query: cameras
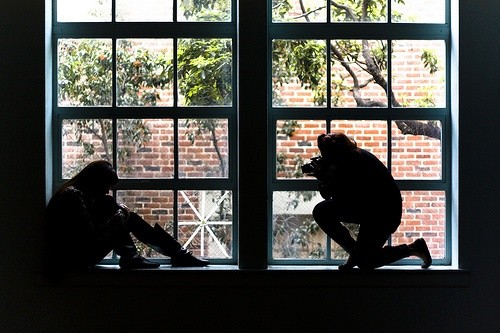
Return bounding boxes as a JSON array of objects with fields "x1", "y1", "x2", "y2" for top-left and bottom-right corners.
[{"x1": 301, "y1": 156, "x2": 322, "y2": 177}]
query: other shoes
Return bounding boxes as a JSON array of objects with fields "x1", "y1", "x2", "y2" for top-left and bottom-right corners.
[
  {"x1": 339, "y1": 250, "x2": 359, "y2": 271},
  {"x1": 413, "y1": 240, "x2": 433, "y2": 270}
]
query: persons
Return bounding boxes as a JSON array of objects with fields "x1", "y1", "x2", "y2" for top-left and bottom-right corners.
[
  {"x1": 313, "y1": 133, "x2": 433, "y2": 270},
  {"x1": 46, "y1": 161, "x2": 210, "y2": 270}
]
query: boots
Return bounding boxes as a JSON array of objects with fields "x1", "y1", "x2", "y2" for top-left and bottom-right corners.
[
  {"x1": 111, "y1": 225, "x2": 160, "y2": 268},
  {"x1": 146, "y1": 223, "x2": 210, "y2": 267}
]
